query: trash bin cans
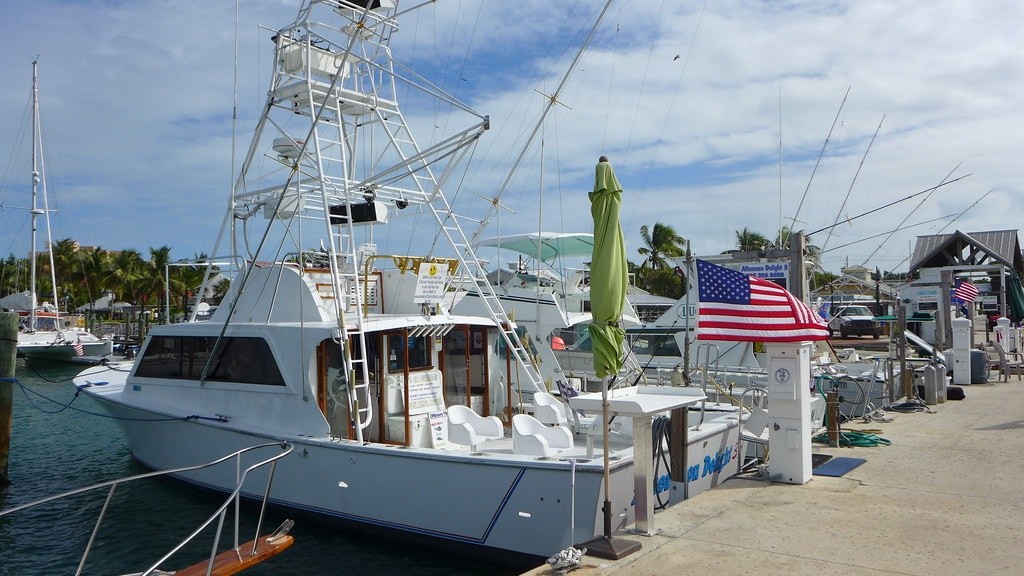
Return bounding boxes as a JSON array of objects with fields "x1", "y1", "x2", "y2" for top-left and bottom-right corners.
[{"x1": 971, "y1": 349, "x2": 987, "y2": 384}]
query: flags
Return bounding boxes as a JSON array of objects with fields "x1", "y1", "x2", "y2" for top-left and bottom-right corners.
[
  {"x1": 954, "y1": 278, "x2": 979, "y2": 304},
  {"x1": 74, "y1": 343, "x2": 83, "y2": 357},
  {"x1": 695, "y1": 258, "x2": 831, "y2": 342}
]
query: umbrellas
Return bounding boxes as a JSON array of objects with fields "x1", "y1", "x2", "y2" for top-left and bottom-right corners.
[
  {"x1": 589, "y1": 161, "x2": 629, "y2": 538},
  {"x1": 1007, "y1": 268, "x2": 1024, "y2": 360}
]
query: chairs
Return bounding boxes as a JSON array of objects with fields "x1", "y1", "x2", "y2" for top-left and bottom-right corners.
[
  {"x1": 571, "y1": 413, "x2": 605, "y2": 459},
  {"x1": 981, "y1": 341, "x2": 1013, "y2": 380},
  {"x1": 445, "y1": 404, "x2": 505, "y2": 453},
  {"x1": 991, "y1": 340, "x2": 1024, "y2": 383},
  {"x1": 533, "y1": 392, "x2": 573, "y2": 428},
  {"x1": 512, "y1": 414, "x2": 574, "y2": 458}
]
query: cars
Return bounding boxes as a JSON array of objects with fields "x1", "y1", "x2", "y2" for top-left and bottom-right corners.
[{"x1": 829, "y1": 302, "x2": 883, "y2": 339}]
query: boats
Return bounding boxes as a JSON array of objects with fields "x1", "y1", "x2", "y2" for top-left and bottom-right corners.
[
  {"x1": 18, "y1": 306, "x2": 114, "y2": 367},
  {"x1": 72, "y1": 0, "x2": 750, "y2": 562},
  {"x1": 436, "y1": 262, "x2": 904, "y2": 459}
]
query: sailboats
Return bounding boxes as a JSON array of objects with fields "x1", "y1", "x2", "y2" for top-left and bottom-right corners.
[{"x1": 0, "y1": 54, "x2": 117, "y2": 371}]
